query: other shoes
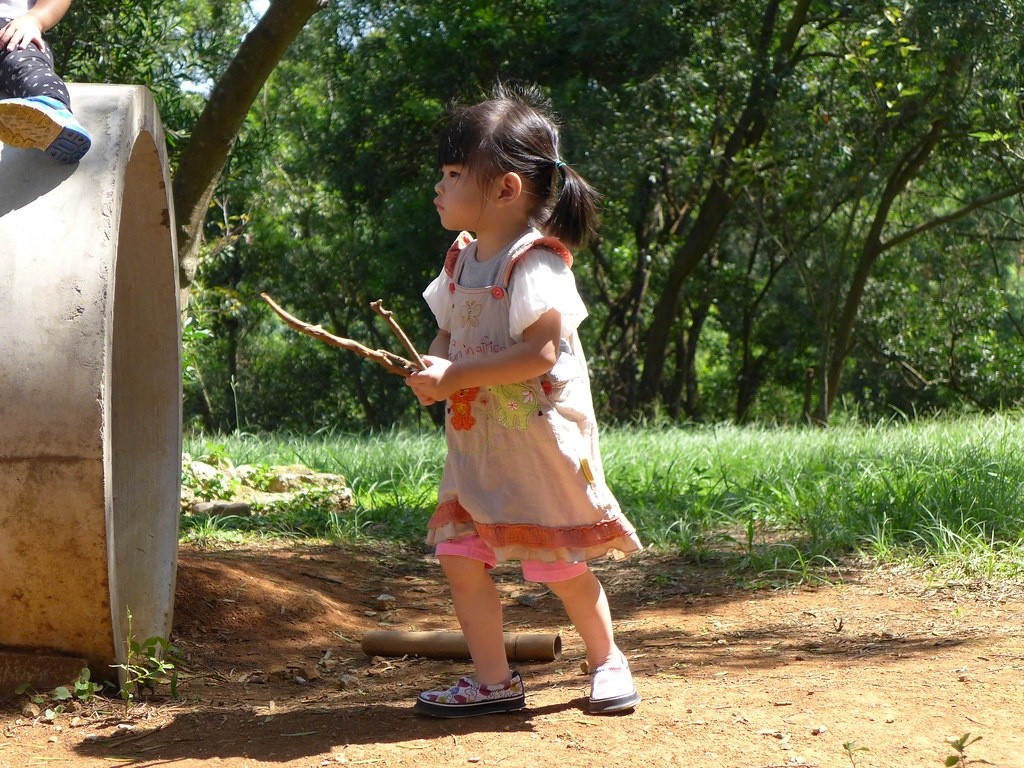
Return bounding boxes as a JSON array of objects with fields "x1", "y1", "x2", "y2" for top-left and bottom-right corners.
[
  {"x1": 587, "y1": 653, "x2": 640, "y2": 714},
  {"x1": 416, "y1": 671, "x2": 527, "y2": 718},
  {"x1": 0, "y1": 95, "x2": 91, "y2": 165}
]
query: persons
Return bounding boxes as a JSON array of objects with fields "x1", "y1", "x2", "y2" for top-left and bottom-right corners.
[
  {"x1": 407, "y1": 80, "x2": 643, "y2": 719},
  {"x1": 0, "y1": 0, "x2": 91, "y2": 164}
]
